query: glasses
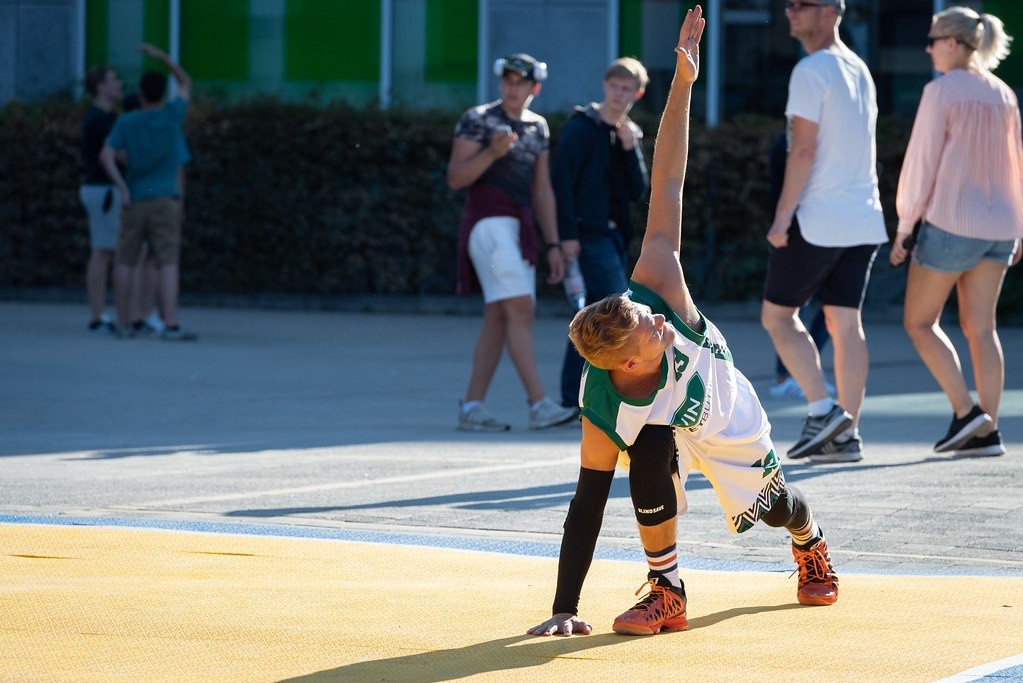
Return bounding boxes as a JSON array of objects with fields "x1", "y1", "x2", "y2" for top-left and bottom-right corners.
[
  {"x1": 785, "y1": 2, "x2": 828, "y2": 11},
  {"x1": 928, "y1": 35, "x2": 955, "y2": 47}
]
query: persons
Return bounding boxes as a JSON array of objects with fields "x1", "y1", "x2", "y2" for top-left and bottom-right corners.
[
  {"x1": 889, "y1": 5, "x2": 1023, "y2": 457},
  {"x1": 99, "y1": 42, "x2": 200, "y2": 341},
  {"x1": 443, "y1": 52, "x2": 577, "y2": 431},
  {"x1": 548, "y1": 53, "x2": 648, "y2": 413},
  {"x1": 765, "y1": 127, "x2": 836, "y2": 402},
  {"x1": 69, "y1": 62, "x2": 124, "y2": 332},
  {"x1": 526, "y1": 5, "x2": 839, "y2": 641},
  {"x1": 758, "y1": 0, "x2": 889, "y2": 462}
]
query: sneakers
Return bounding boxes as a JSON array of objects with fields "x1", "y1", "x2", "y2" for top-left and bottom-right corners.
[
  {"x1": 809, "y1": 438, "x2": 862, "y2": 461},
  {"x1": 527, "y1": 398, "x2": 579, "y2": 431},
  {"x1": 956, "y1": 430, "x2": 1006, "y2": 455},
  {"x1": 769, "y1": 377, "x2": 836, "y2": 400},
  {"x1": 934, "y1": 404, "x2": 991, "y2": 452},
  {"x1": 787, "y1": 405, "x2": 852, "y2": 459},
  {"x1": 458, "y1": 399, "x2": 511, "y2": 432},
  {"x1": 613, "y1": 570, "x2": 688, "y2": 635},
  {"x1": 789, "y1": 526, "x2": 838, "y2": 605}
]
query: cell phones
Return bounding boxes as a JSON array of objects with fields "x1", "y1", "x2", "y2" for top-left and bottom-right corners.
[{"x1": 496, "y1": 125, "x2": 513, "y2": 136}]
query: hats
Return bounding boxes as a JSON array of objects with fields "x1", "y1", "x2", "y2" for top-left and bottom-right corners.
[{"x1": 493, "y1": 53, "x2": 547, "y2": 80}]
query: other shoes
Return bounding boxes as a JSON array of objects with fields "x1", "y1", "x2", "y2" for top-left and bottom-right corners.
[{"x1": 86, "y1": 319, "x2": 197, "y2": 340}]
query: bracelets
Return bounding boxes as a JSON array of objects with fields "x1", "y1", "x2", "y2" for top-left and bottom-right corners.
[{"x1": 549, "y1": 242, "x2": 562, "y2": 250}]
